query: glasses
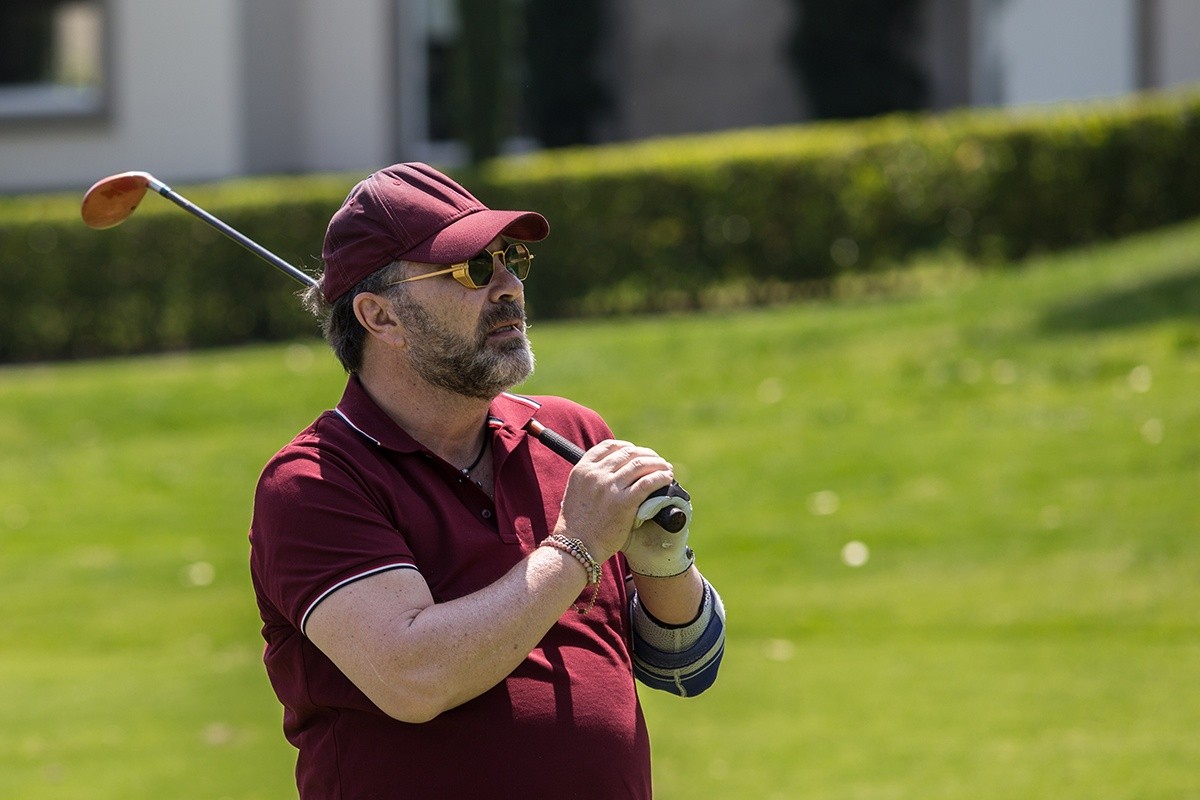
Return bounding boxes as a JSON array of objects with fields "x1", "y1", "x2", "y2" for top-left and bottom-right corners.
[{"x1": 368, "y1": 243, "x2": 534, "y2": 295}]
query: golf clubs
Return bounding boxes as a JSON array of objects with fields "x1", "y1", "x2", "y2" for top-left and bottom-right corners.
[{"x1": 80, "y1": 170, "x2": 688, "y2": 534}]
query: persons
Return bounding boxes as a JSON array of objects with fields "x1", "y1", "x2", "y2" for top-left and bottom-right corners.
[{"x1": 247, "y1": 161, "x2": 725, "y2": 800}]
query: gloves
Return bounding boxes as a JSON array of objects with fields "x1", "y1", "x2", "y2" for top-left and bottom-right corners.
[{"x1": 619, "y1": 478, "x2": 696, "y2": 577}]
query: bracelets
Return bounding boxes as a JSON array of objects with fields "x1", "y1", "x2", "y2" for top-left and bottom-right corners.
[{"x1": 540, "y1": 534, "x2": 603, "y2": 616}]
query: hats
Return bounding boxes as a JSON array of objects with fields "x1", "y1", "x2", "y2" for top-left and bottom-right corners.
[{"x1": 322, "y1": 162, "x2": 549, "y2": 305}]
